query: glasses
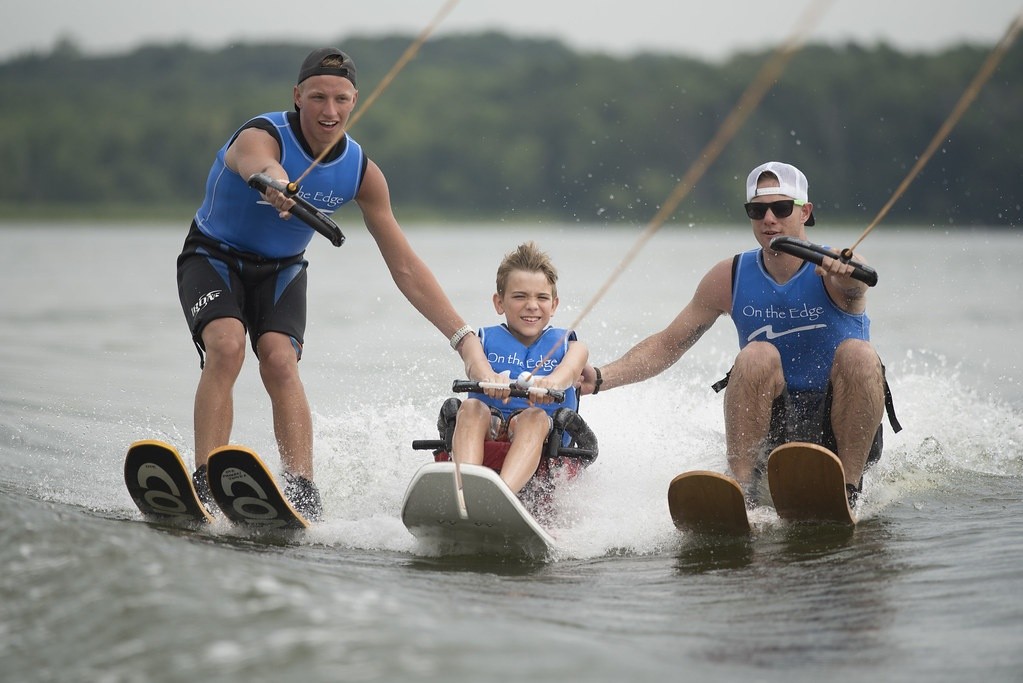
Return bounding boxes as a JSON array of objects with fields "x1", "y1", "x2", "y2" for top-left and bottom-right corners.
[{"x1": 744, "y1": 200, "x2": 807, "y2": 220}]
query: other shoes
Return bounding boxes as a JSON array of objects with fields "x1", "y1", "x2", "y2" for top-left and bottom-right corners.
[
  {"x1": 192, "y1": 464, "x2": 221, "y2": 520},
  {"x1": 280, "y1": 472, "x2": 322, "y2": 522}
]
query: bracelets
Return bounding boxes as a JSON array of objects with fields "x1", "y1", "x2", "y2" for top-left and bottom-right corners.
[{"x1": 593, "y1": 367, "x2": 603, "y2": 396}]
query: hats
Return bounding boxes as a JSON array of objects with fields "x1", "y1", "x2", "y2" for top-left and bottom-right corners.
[
  {"x1": 295, "y1": 47, "x2": 357, "y2": 113},
  {"x1": 746, "y1": 162, "x2": 815, "y2": 226}
]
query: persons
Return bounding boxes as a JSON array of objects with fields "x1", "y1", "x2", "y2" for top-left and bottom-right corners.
[
  {"x1": 450, "y1": 245, "x2": 587, "y2": 497},
  {"x1": 176, "y1": 45, "x2": 476, "y2": 521},
  {"x1": 577, "y1": 162, "x2": 902, "y2": 514}
]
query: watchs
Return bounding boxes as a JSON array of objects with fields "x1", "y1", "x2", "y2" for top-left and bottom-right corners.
[{"x1": 451, "y1": 322, "x2": 475, "y2": 349}]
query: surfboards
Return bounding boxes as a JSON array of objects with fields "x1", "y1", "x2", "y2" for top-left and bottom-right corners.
[
  {"x1": 123, "y1": 439, "x2": 313, "y2": 531},
  {"x1": 668, "y1": 441, "x2": 857, "y2": 535},
  {"x1": 400, "y1": 461, "x2": 557, "y2": 552}
]
